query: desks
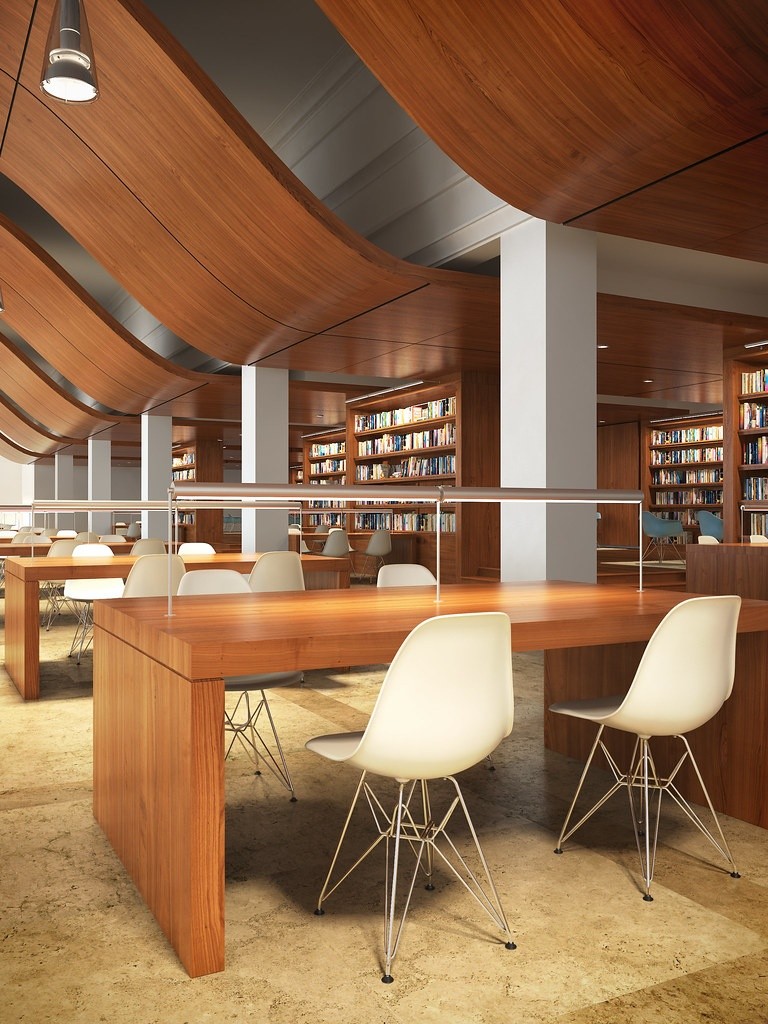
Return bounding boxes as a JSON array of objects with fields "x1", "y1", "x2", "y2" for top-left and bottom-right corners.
[
  {"x1": 302, "y1": 532, "x2": 420, "y2": 585},
  {"x1": 4, "y1": 552, "x2": 351, "y2": 701},
  {"x1": 0, "y1": 541, "x2": 184, "y2": 556},
  {"x1": 0, "y1": 537, "x2": 75, "y2": 543},
  {"x1": 685, "y1": 542, "x2": 768, "y2": 601},
  {"x1": 93, "y1": 579, "x2": 767, "y2": 978}
]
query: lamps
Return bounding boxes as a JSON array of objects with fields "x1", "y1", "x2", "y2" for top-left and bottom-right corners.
[
  {"x1": 37, "y1": 0, "x2": 100, "y2": 105},
  {"x1": 167, "y1": 480, "x2": 442, "y2": 503},
  {"x1": 0, "y1": 500, "x2": 301, "y2": 513},
  {"x1": 345, "y1": 380, "x2": 440, "y2": 403},
  {"x1": 438, "y1": 487, "x2": 645, "y2": 504}
]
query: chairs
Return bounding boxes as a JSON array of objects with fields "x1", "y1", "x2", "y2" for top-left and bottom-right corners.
[
  {"x1": 697, "y1": 511, "x2": 724, "y2": 541},
  {"x1": 641, "y1": 511, "x2": 686, "y2": 565},
  {"x1": 0, "y1": 522, "x2": 767, "y2": 987}
]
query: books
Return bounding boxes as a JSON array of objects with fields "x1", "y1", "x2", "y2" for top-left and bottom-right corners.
[
  {"x1": 741, "y1": 368, "x2": 768, "y2": 394},
  {"x1": 308, "y1": 500, "x2": 346, "y2": 527},
  {"x1": 744, "y1": 512, "x2": 768, "y2": 539},
  {"x1": 172, "y1": 454, "x2": 195, "y2": 525},
  {"x1": 310, "y1": 442, "x2": 346, "y2": 486},
  {"x1": 296, "y1": 470, "x2": 303, "y2": 484},
  {"x1": 740, "y1": 402, "x2": 768, "y2": 429},
  {"x1": 650, "y1": 426, "x2": 723, "y2": 544},
  {"x1": 355, "y1": 484, "x2": 455, "y2": 532},
  {"x1": 742, "y1": 436, "x2": 768, "y2": 465},
  {"x1": 355, "y1": 396, "x2": 456, "y2": 480},
  {"x1": 742, "y1": 476, "x2": 768, "y2": 500}
]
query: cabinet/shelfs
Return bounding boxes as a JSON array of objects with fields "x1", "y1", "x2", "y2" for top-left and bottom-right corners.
[
  {"x1": 303, "y1": 429, "x2": 346, "y2": 554},
  {"x1": 721, "y1": 344, "x2": 768, "y2": 542},
  {"x1": 648, "y1": 414, "x2": 723, "y2": 561},
  {"x1": 344, "y1": 367, "x2": 501, "y2": 585},
  {"x1": 291, "y1": 468, "x2": 303, "y2": 517},
  {"x1": 172, "y1": 440, "x2": 224, "y2": 543}
]
